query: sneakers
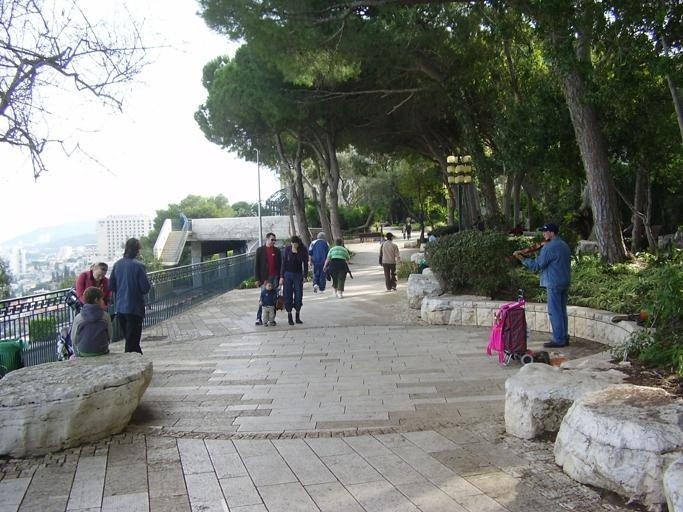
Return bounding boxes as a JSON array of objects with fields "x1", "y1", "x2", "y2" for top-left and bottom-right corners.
[{"x1": 256, "y1": 319, "x2": 275, "y2": 326}]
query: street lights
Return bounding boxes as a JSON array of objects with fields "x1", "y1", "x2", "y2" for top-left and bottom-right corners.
[
  {"x1": 444, "y1": 151, "x2": 474, "y2": 227},
  {"x1": 253, "y1": 144, "x2": 265, "y2": 249}
]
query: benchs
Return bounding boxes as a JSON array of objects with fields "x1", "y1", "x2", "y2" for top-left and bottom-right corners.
[{"x1": 359, "y1": 232, "x2": 384, "y2": 244}]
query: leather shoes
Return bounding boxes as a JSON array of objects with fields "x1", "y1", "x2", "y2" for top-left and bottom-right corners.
[{"x1": 544, "y1": 343, "x2": 564, "y2": 347}]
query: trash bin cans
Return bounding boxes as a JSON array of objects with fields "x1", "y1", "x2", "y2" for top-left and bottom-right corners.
[{"x1": 0, "y1": 339, "x2": 25, "y2": 376}]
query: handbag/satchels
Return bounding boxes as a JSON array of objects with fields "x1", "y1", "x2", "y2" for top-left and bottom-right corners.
[{"x1": 276, "y1": 296, "x2": 284, "y2": 309}]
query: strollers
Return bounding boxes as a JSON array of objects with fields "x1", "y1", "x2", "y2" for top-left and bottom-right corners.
[{"x1": 55, "y1": 312, "x2": 117, "y2": 361}]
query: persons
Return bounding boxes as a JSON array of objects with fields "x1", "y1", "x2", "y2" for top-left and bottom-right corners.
[
  {"x1": 513, "y1": 224, "x2": 571, "y2": 347},
  {"x1": 379, "y1": 233, "x2": 400, "y2": 290},
  {"x1": 253, "y1": 233, "x2": 281, "y2": 325},
  {"x1": 109, "y1": 238, "x2": 151, "y2": 354},
  {"x1": 75, "y1": 262, "x2": 114, "y2": 312},
  {"x1": 323, "y1": 239, "x2": 353, "y2": 298},
  {"x1": 71, "y1": 287, "x2": 112, "y2": 357},
  {"x1": 259, "y1": 280, "x2": 281, "y2": 326},
  {"x1": 279, "y1": 236, "x2": 308, "y2": 325},
  {"x1": 406, "y1": 216, "x2": 412, "y2": 239},
  {"x1": 309, "y1": 232, "x2": 329, "y2": 293}
]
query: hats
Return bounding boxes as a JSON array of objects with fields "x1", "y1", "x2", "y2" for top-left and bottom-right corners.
[{"x1": 539, "y1": 223, "x2": 557, "y2": 232}]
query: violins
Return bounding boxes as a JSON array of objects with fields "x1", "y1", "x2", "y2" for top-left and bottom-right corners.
[{"x1": 503, "y1": 240, "x2": 548, "y2": 263}]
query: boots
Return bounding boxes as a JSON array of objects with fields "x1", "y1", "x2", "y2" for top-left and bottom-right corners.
[
  {"x1": 288, "y1": 314, "x2": 293, "y2": 325},
  {"x1": 296, "y1": 313, "x2": 302, "y2": 323}
]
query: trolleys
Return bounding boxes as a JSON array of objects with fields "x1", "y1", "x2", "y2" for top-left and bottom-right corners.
[{"x1": 490, "y1": 286, "x2": 534, "y2": 367}]
